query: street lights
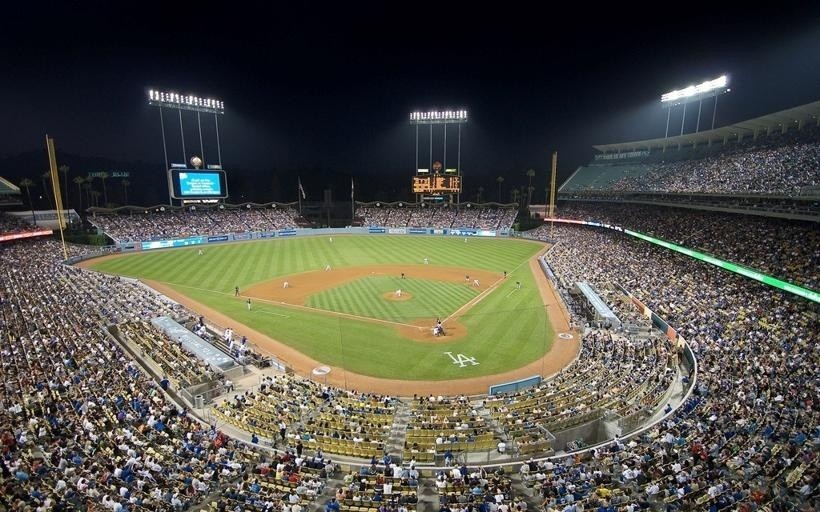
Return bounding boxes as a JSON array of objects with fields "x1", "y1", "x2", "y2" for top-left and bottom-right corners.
[
  {"x1": 409, "y1": 110, "x2": 468, "y2": 204},
  {"x1": 146, "y1": 89, "x2": 226, "y2": 205},
  {"x1": 660, "y1": 74, "x2": 729, "y2": 138}
]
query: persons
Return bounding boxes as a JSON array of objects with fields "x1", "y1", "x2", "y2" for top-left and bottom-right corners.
[
  {"x1": 503, "y1": 270, "x2": 507, "y2": 278},
  {"x1": 516, "y1": 281, "x2": 521, "y2": 290},
  {"x1": 207, "y1": 318, "x2": 547, "y2": 512},
  {"x1": 395, "y1": 287, "x2": 402, "y2": 297},
  {"x1": 401, "y1": 272, "x2": 406, "y2": 279},
  {"x1": 234, "y1": 286, "x2": 241, "y2": 296},
  {"x1": 325, "y1": 264, "x2": 332, "y2": 272},
  {"x1": 465, "y1": 275, "x2": 470, "y2": 287},
  {"x1": 472, "y1": 279, "x2": 480, "y2": 287},
  {"x1": 355, "y1": 199, "x2": 548, "y2": 241},
  {"x1": 0, "y1": 204, "x2": 207, "y2": 511},
  {"x1": 207, "y1": 205, "x2": 302, "y2": 238},
  {"x1": 547, "y1": 129, "x2": 820, "y2": 512},
  {"x1": 246, "y1": 298, "x2": 252, "y2": 311},
  {"x1": 283, "y1": 280, "x2": 288, "y2": 288},
  {"x1": 424, "y1": 256, "x2": 428, "y2": 264}
]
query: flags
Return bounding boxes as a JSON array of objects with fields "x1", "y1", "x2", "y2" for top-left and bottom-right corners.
[{"x1": 299, "y1": 183, "x2": 306, "y2": 199}]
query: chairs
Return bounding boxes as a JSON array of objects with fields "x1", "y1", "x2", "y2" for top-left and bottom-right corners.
[{"x1": 0, "y1": 272, "x2": 820, "y2": 512}]
